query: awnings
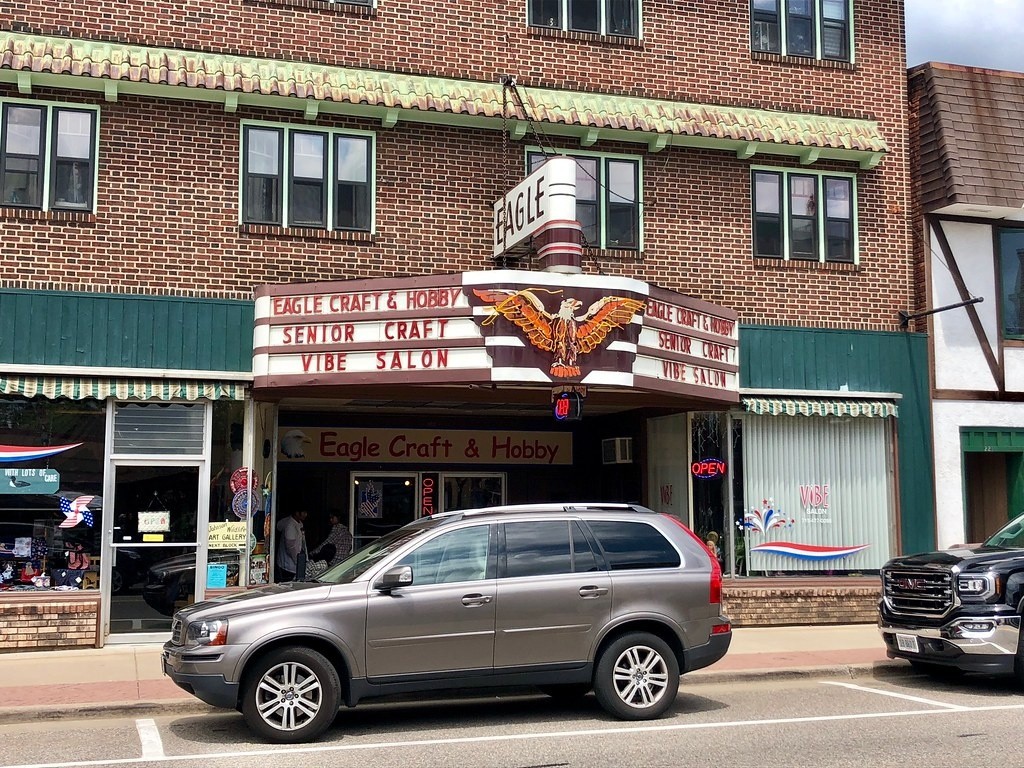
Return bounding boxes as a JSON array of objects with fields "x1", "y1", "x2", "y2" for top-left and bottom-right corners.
[{"x1": 741, "y1": 395, "x2": 898, "y2": 418}]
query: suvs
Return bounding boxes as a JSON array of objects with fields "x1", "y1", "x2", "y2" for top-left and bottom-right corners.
[
  {"x1": 873, "y1": 509, "x2": 1024, "y2": 697},
  {"x1": 158, "y1": 502, "x2": 735, "y2": 746}
]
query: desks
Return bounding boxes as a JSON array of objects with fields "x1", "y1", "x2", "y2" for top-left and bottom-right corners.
[{"x1": 50, "y1": 569, "x2": 97, "y2": 589}]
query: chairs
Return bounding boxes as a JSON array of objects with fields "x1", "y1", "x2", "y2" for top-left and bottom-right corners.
[{"x1": 85, "y1": 556, "x2": 101, "y2": 588}]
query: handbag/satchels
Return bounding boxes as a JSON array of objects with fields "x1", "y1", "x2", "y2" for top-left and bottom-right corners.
[
  {"x1": 68, "y1": 552, "x2": 90, "y2": 569},
  {"x1": 20, "y1": 568, "x2": 40, "y2": 583},
  {"x1": 32, "y1": 573, "x2": 51, "y2": 590},
  {"x1": 137, "y1": 495, "x2": 171, "y2": 532},
  {"x1": 1, "y1": 564, "x2": 17, "y2": 580},
  {"x1": 23, "y1": 561, "x2": 41, "y2": 575}
]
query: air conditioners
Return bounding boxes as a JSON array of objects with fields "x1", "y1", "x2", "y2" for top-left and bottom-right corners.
[{"x1": 602, "y1": 437, "x2": 633, "y2": 464}]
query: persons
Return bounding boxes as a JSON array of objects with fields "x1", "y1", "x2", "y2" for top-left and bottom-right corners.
[
  {"x1": 276, "y1": 503, "x2": 353, "y2": 584},
  {"x1": 706, "y1": 541, "x2": 718, "y2": 556}
]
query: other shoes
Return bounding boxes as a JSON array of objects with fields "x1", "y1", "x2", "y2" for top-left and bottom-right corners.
[{"x1": 72, "y1": 544, "x2": 83, "y2": 551}]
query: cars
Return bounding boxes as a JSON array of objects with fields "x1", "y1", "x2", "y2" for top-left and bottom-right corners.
[
  {"x1": 140, "y1": 537, "x2": 271, "y2": 618},
  {"x1": 0, "y1": 521, "x2": 142, "y2": 597}
]
